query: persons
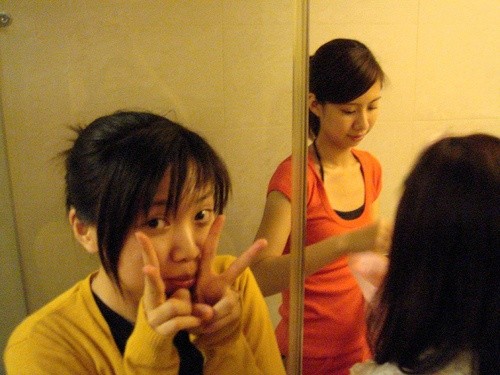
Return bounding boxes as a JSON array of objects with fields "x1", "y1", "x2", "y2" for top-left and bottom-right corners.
[
  {"x1": 249, "y1": 38, "x2": 393, "y2": 375},
  {"x1": 4, "y1": 110, "x2": 287, "y2": 375},
  {"x1": 349, "y1": 133, "x2": 500, "y2": 375}
]
